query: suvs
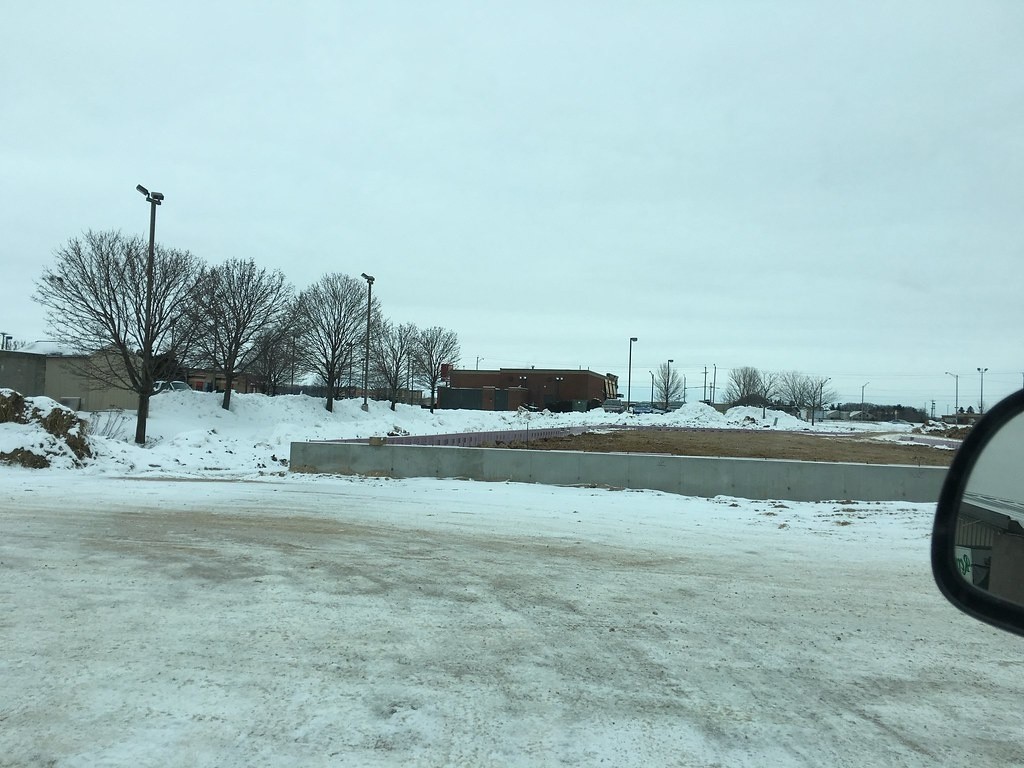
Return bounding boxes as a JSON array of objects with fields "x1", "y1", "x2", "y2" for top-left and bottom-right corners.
[
  {"x1": 602, "y1": 399, "x2": 624, "y2": 414},
  {"x1": 627, "y1": 403, "x2": 636, "y2": 411}
]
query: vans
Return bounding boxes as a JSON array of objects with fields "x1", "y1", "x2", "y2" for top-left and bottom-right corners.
[{"x1": 153, "y1": 380, "x2": 192, "y2": 392}]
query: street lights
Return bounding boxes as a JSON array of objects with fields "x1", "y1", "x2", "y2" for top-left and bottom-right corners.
[
  {"x1": 346, "y1": 342, "x2": 352, "y2": 396},
  {"x1": 361, "y1": 272, "x2": 375, "y2": 412},
  {"x1": 291, "y1": 333, "x2": 301, "y2": 394},
  {"x1": 666, "y1": 360, "x2": 673, "y2": 410},
  {"x1": 6, "y1": 336, "x2": 13, "y2": 350},
  {"x1": 136, "y1": 184, "x2": 164, "y2": 443},
  {"x1": 0, "y1": 332, "x2": 5, "y2": 349},
  {"x1": 628, "y1": 338, "x2": 637, "y2": 411},
  {"x1": 946, "y1": 372, "x2": 958, "y2": 427},
  {"x1": 977, "y1": 368, "x2": 988, "y2": 415}
]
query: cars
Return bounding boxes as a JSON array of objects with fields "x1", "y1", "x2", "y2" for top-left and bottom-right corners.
[
  {"x1": 421, "y1": 398, "x2": 437, "y2": 409},
  {"x1": 633, "y1": 403, "x2": 653, "y2": 414}
]
